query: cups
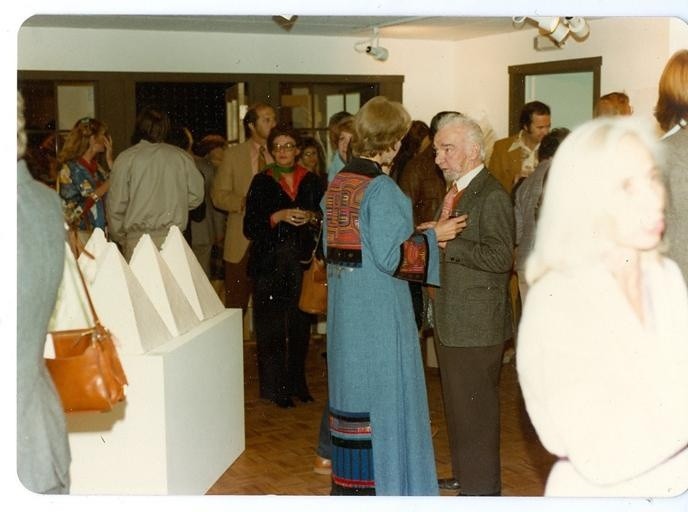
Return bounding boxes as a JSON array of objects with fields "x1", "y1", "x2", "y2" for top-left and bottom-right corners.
[{"x1": 448, "y1": 209, "x2": 463, "y2": 220}]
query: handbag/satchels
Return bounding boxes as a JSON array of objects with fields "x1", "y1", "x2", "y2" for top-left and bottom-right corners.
[
  {"x1": 43, "y1": 323, "x2": 128, "y2": 414},
  {"x1": 297, "y1": 259, "x2": 329, "y2": 316}
]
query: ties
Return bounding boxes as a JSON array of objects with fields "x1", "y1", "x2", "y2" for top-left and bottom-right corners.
[
  {"x1": 428, "y1": 184, "x2": 457, "y2": 300},
  {"x1": 257, "y1": 146, "x2": 267, "y2": 171}
]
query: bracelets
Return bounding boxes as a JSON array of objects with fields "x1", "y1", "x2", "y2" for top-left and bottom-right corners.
[{"x1": 90, "y1": 192, "x2": 100, "y2": 202}]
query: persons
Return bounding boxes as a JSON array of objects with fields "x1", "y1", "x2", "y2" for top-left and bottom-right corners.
[
  {"x1": 488, "y1": 100, "x2": 572, "y2": 304},
  {"x1": 647, "y1": 46, "x2": 686, "y2": 284},
  {"x1": 511, "y1": 116, "x2": 687, "y2": 497},
  {"x1": 313, "y1": 96, "x2": 514, "y2": 495},
  {"x1": 16, "y1": 90, "x2": 74, "y2": 494},
  {"x1": 170, "y1": 104, "x2": 354, "y2": 411},
  {"x1": 54, "y1": 115, "x2": 115, "y2": 259},
  {"x1": 590, "y1": 90, "x2": 634, "y2": 118},
  {"x1": 104, "y1": 102, "x2": 206, "y2": 264}
]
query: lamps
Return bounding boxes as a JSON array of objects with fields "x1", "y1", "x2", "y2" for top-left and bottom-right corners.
[
  {"x1": 512, "y1": 16, "x2": 590, "y2": 44},
  {"x1": 353, "y1": 26, "x2": 389, "y2": 62}
]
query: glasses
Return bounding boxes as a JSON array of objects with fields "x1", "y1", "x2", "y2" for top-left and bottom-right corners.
[
  {"x1": 271, "y1": 142, "x2": 298, "y2": 153},
  {"x1": 302, "y1": 151, "x2": 321, "y2": 160}
]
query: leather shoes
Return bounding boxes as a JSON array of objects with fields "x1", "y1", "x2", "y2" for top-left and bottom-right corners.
[{"x1": 437, "y1": 478, "x2": 460, "y2": 490}]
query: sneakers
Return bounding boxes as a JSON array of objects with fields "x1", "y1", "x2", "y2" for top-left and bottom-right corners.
[{"x1": 313, "y1": 453, "x2": 333, "y2": 476}]
